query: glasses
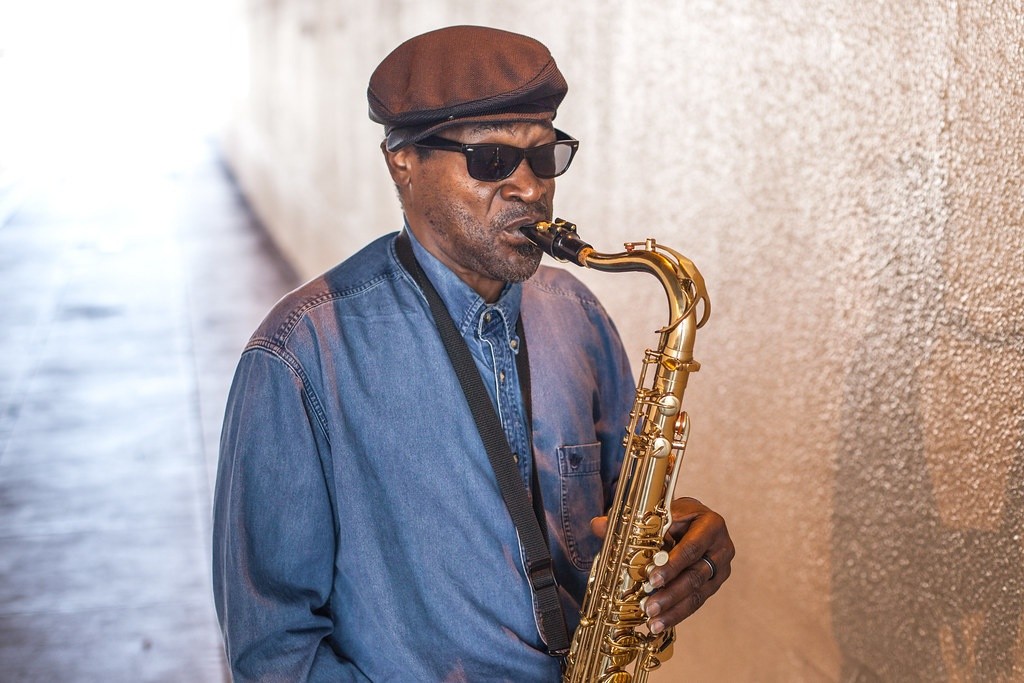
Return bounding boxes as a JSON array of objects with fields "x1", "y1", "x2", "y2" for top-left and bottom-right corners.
[{"x1": 412, "y1": 128, "x2": 579, "y2": 184}]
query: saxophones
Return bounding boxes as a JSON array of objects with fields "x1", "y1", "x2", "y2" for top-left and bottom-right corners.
[{"x1": 516, "y1": 214, "x2": 712, "y2": 683}]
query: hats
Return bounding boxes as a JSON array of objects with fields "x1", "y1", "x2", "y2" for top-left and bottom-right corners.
[{"x1": 365, "y1": 23, "x2": 570, "y2": 130}]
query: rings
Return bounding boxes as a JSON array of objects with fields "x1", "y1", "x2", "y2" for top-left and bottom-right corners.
[{"x1": 701, "y1": 554, "x2": 718, "y2": 581}]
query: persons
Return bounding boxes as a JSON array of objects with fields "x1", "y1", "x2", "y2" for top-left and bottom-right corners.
[{"x1": 212, "y1": 24, "x2": 737, "y2": 682}]
